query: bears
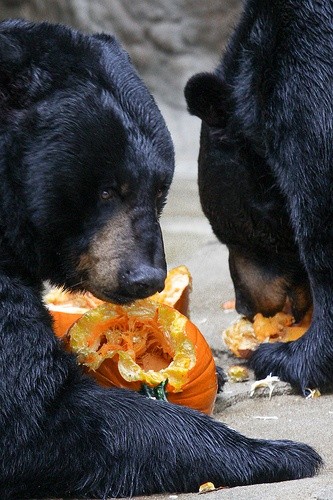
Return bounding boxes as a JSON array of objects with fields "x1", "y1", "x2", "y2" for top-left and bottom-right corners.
[
  {"x1": 0, "y1": 19, "x2": 323, "y2": 500},
  {"x1": 182, "y1": 0, "x2": 333, "y2": 398}
]
